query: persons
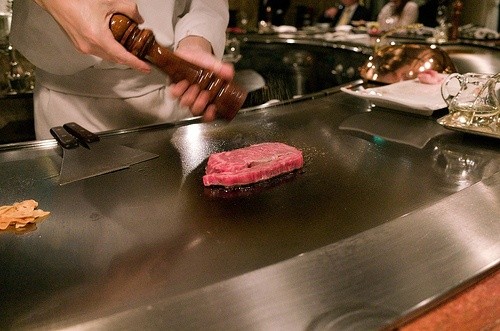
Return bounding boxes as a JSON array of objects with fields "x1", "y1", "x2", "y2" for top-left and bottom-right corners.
[
  {"x1": 317, "y1": 0, "x2": 370, "y2": 30},
  {"x1": 256, "y1": 0, "x2": 290, "y2": 27},
  {"x1": 8, "y1": 0, "x2": 235, "y2": 141},
  {"x1": 376, "y1": 0, "x2": 419, "y2": 30}
]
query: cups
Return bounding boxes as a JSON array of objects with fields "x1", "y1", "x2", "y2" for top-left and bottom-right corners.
[{"x1": 441, "y1": 72, "x2": 500, "y2": 128}]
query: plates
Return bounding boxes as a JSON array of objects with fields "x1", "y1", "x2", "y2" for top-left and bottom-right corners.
[{"x1": 339, "y1": 72, "x2": 459, "y2": 115}]
